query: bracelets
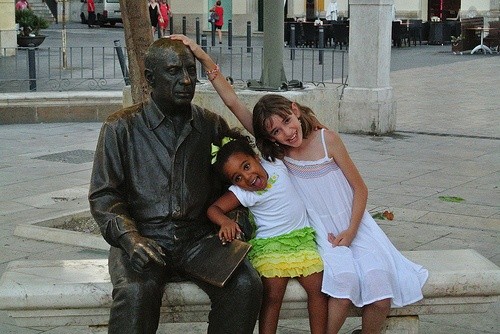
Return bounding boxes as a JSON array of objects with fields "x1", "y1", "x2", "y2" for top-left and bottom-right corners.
[{"x1": 207, "y1": 65, "x2": 220, "y2": 81}]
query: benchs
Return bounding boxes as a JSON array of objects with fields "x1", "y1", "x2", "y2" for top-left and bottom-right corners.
[{"x1": 0, "y1": 248, "x2": 500, "y2": 334}]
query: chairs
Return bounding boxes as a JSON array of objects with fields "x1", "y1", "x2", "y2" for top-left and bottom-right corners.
[{"x1": 284, "y1": 18, "x2": 422, "y2": 50}]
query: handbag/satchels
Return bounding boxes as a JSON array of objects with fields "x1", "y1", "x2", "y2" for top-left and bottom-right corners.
[{"x1": 208, "y1": 11, "x2": 220, "y2": 22}]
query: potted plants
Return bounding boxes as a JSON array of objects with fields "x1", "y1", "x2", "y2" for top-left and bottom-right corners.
[
  {"x1": 451, "y1": 34, "x2": 465, "y2": 53},
  {"x1": 15, "y1": 8, "x2": 47, "y2": 48}
]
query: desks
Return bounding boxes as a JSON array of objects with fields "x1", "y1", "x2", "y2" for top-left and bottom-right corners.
[
  {"x1": 400, "y1": 23, "x2": 415, "y2": 47},
  {"x1": 465, "y1": 27, "x2": 500, "y2": 55},
  {"x1": 323, "y1": 24, "x2": 334, "y2": 47}
]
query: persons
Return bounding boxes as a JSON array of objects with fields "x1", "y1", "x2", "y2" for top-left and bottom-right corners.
[
  {"x1": 209, "y1": 1, "x2": 223, "y2": 44},
  {"x1": 87, "y1": 0, "x2": 95, "y2": 29},
  {"x1": 88, "y1": 38, "x2": 263, "y2": 332},
  {"x1": 147, "y1": 0, "x2": 163, "y2": 36},
  {"x1": 157, "y1": 0, "x2": 173, "y2": 37},
  {"x1": 16, "y1": 0, "x2": 29, "y2": 14},
  {"x1": 165, "y1": 34, "x2": 394, "y2": 334},
  {"x1": 206, "y1": 136, "x2": 329, "y2": 334}
]
